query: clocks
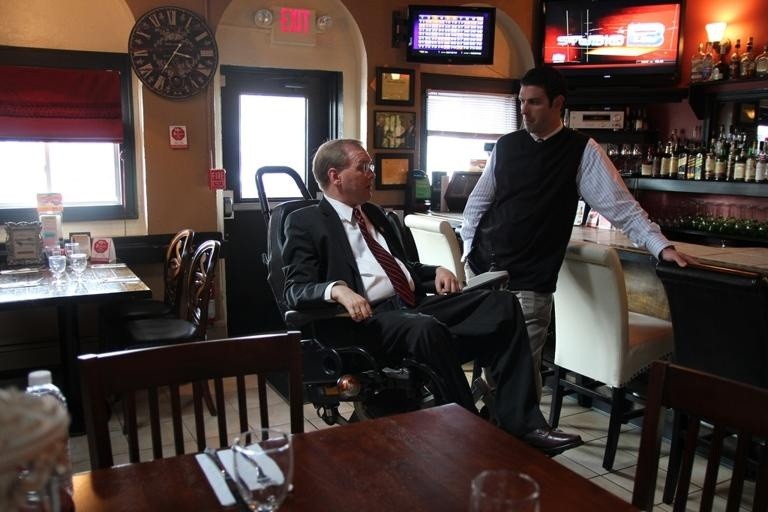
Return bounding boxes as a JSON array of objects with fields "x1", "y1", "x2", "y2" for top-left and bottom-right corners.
[{"x1": 127, "y1": 5, "x2": 220, "y2": 102}]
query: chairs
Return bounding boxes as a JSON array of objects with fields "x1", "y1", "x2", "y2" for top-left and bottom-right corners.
[
  {"x1": 404, "y1": 212, "x2": 511, "y2": 294},
  {"x1": 108, "y1": 240, "x2": 221, "y2": 433},
  {"x1": 121, "y1": 229, "x2": 195, "y2": 319},
  {"x1": 548, "y1": 241, "x2": 677, "y2": 470},
  {"x1": 78, "y1": 331, "x2": 303, "y2": 465},
  {"x1": 631, "y1": 360, "x2": 768, "y2": 512}
]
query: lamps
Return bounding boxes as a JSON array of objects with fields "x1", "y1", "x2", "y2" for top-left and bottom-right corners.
[
  {"x1": 705, "y1": 22, "x2": 727, "y2": 44},
  {"x1": 555, "y1": 8, "x2": 627, "y2": 47}
]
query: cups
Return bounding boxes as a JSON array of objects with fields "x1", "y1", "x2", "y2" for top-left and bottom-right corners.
[
  {"x1": 49, "y1": 255, "x2": 66, "y2": 292},
  {"x1": 64, "y1": 243, "x2": 80, "y2": 274},
  {"x1": 469, "y1": 469, "x2": 540, "y2": 512},
  {"x1": 45, "y1": 245, "x2": 61, "y2": 258},
  {"x1": 70, "y1": 254, "x2": 88, "y2": 294},
  {"x1": 231, "y1": 427, "x2": 296, "y2": 512}
]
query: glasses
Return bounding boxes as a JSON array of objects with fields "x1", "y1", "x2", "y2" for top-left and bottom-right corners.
[{"x1": 336, "y1": 160, "x2": 376, "y2": 174}]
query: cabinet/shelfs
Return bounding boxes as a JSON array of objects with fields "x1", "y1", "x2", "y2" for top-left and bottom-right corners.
[{"x1": 398, "y1": 0, "x2": 768, "y2": 436}]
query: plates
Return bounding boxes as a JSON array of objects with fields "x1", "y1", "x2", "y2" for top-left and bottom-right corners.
[{"x1": 194, "y1": 442, "x2": 294, "y2": 507}]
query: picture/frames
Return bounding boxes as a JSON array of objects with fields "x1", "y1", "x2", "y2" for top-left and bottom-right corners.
[
  {"x1": 376, "y1": 67, "x2": 415, "y2": 105},
  {"x1": 375, "y1": 154, "x2": 413, "y2": 191},
  {"x1": 374, "y1": 111, "x2": 417, "y2": 148}
]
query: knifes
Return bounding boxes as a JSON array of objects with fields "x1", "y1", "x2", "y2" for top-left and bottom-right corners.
[{"x1": 203, "y1": 445, "x2": 253, "y2": 511}]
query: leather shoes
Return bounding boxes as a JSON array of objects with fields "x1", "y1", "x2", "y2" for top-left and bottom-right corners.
[{"x1": 521, "y1": 427, "x2": 584, "y2": 454}]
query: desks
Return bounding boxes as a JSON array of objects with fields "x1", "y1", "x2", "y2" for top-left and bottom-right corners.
[
  {"x1": 0, "y1": 251, "x2": 151, "y2": 400},
  {"x1": 67, "y1": 403, "x2": 645, "y2": 512}
]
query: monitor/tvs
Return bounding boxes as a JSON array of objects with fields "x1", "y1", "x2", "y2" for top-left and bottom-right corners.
[
  {"x1": 537, "y1": 2, "x2": 686, "y2": 79},
  {"x1": 403, "y1": 5, "x2": 496, "y2": 65}
]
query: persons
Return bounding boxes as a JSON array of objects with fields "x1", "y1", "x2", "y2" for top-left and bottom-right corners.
[
  {"x1": 459, "y1": 67, "x2": 701, "y2": 423},
  {"x1": 283, "y1": 140, "x2": 585, "y2": 454}
]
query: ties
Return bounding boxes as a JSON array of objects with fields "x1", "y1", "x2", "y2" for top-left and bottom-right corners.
[{"x1": 352, "y1": 207, "x2": 417, "y2": 309}]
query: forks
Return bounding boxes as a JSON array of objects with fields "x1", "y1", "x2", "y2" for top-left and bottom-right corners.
[{"x1": 232, "y1": 445, "x2": 283, "y2": 497}]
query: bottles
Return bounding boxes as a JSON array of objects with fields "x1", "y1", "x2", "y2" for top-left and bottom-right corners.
[
  {"x1": 660, "y1": 143, "x2": 671, "y2": 177},
  {"x1": 734, "y1": 148, "x2": 744, "y2": 183},
  {"x1": 635, "y1": 109, "x2": 644, "y2": 177},
  {"x1": 669, "y1": 150, "x2": 677, "y2": 178},
  {"x1": 741, "y1": 37, "x2": 756, "y2": 80},
  {"x1": 653, "y1": 141, "x2": 662, "y2": 177},
  {"x1": 640, "y1": 146, "x2": 653, "y2": 177},
  {"x1": 711, "y1": 42, "x2": 729, "y2": 84},
  {"x1": 729, "y1": 39, "x2": 741, "y2": 82},
  {"x1": 702, "y1": 42, "x2": 714, "y2": 83},
  {"x1": 715, "y1": 148, "x2": 726, "y2": 181},
  {"x1": 690, "y1": 43, "x2": 705, "y2": 84},
  {"x1": 687, "y1": 146, "x2": 695, "y2": 180},
  {"x1": 678, "y1": 145, "x2": 688, "y2": 179},
  {"x1": 745, "y1": 147, "x2": 756, "y2": 183},
  {"x1": 755, "y1": 152, "x2": 768, "y2": 183},
  {"x1": 705, "y1": 149, "x2": 715, "y2": 180},
  {"x1": 755, "y1": 45, "x2": 768, "y2": 79},
  {"x1": 664, "y1": 124, "x2": 768, "y2": 150},
  {"x1": 607, "y1": 128, "x2": 621, "y2": 174},
  {"x1": 622, "y1": 107, "x2": 634, "y2": 177},
  {"x1": 24, "y1": 370, "x2": 74, "y2": 510},
  {"x1": 725, "y1": 144, "x2": 734, "y2": 181},
  {"x1": 695, "y1": 146, "x2": 704, "y2": 181}
]
